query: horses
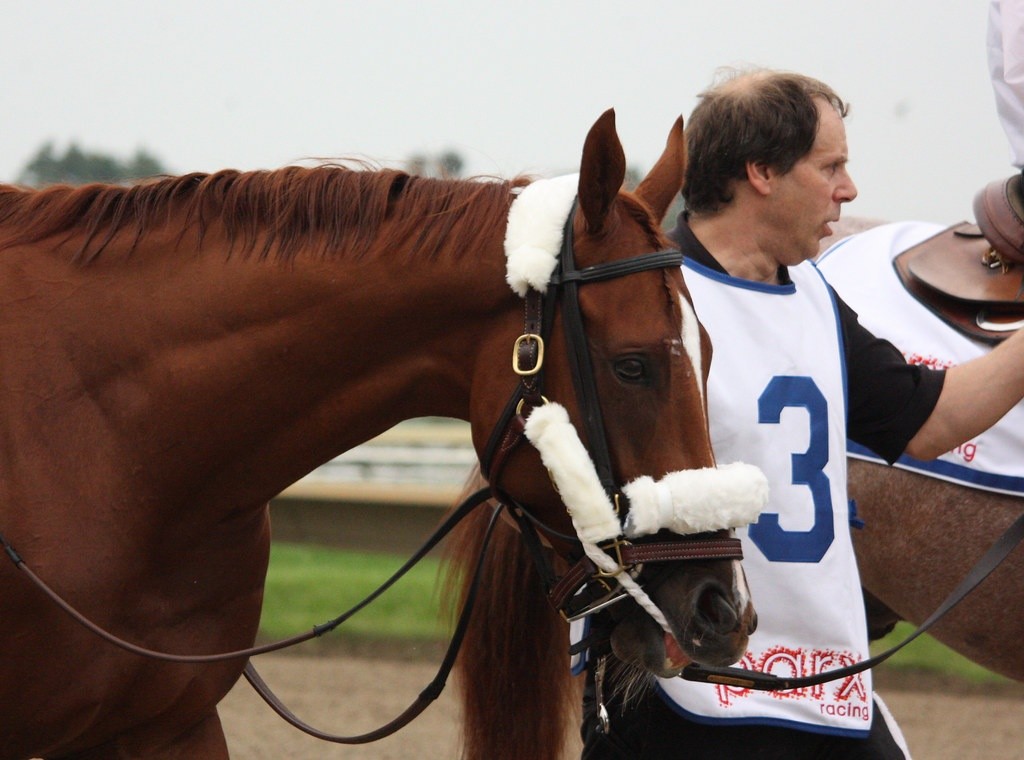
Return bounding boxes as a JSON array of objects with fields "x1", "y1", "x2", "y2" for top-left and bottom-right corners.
[
  {"x1": 0, "y1": 110, "x2": 757, "y2": 759},
  {"x1": 430, "y1": 215, "x2": 1023, "y2": 760}
]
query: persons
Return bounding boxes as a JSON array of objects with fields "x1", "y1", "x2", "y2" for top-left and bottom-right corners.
[
  {"x1": 566, "y1": 65, "x2": 1023, "y2": 760},
  {"x1": 404, "y1": 146, "x2": 463, "y2": 180}
]
